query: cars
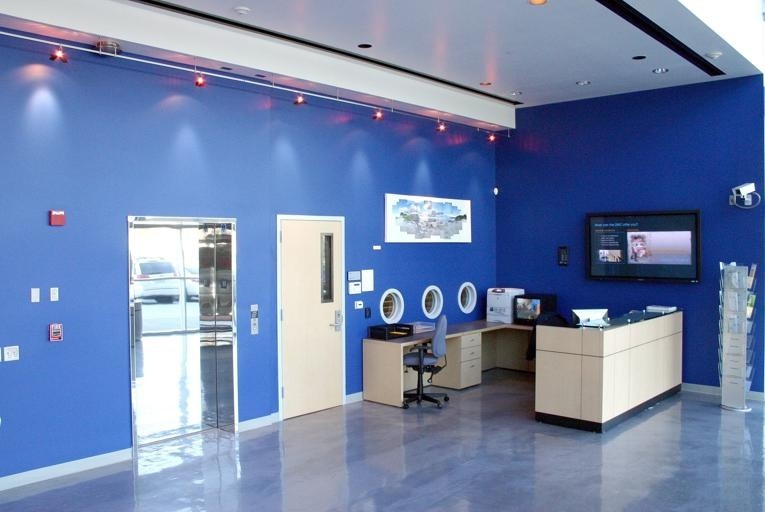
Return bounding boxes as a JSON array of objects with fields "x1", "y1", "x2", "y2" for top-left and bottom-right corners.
[{"x1": 130, "y1": 256, "x2": 199, "y2": 302}]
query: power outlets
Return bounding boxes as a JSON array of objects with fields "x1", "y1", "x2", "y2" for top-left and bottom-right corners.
[{"x1": 3, "y1": 345, "x2": 19, "y2": 362}]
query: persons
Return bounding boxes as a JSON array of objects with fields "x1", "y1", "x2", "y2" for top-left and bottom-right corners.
[{"x1": 630, "y1": 236, "x2": 641, "y2": 261}]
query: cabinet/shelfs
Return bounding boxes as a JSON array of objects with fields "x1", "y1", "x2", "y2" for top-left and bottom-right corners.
[
  {"x1": 361, "y1": 324, "x2": 533, "y2": 408},
  {"x1": 534, "y1": 308, "x2": 684, "y2": 433}
]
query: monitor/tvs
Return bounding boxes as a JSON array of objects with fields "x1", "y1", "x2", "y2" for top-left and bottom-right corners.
[
  {"x1": 586, "y1": 208, "x2": 702, "y2": 288},
  {"x1": 514, "y1": 294, "x2": 557, "y2": 326}
]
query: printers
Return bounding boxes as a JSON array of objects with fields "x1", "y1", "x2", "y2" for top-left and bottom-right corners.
[{"x1": 486, "y1": 287, "x2": 524, "y2": 324}]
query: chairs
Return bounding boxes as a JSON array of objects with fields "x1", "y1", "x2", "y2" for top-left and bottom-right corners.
[
  {"x1": 537, "y1": 314, "x2": 570, "y2": 328},
  {"x1": 401, "y1": 314, "x2": 451, "y2": 411}
]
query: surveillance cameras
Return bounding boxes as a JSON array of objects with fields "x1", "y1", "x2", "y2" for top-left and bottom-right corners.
[{"x1": 732, "y1": 183, "x2": 756, "y2": 197}]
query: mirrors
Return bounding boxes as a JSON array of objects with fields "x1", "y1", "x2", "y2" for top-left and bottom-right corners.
[{"x1": 125, "y1": 214, "x2": 242, "y2": 464}]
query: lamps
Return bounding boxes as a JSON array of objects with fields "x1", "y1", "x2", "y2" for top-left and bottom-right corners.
[
  {"x1": 193, "y1": 73, "x2": 208, "y2": 89},
  {"x1": 486, "y1": 132, "x2": 496, "y2": 144},
  {"x1": 372, "y1": 107, "x2": 385, "y2": 121},
  {"x1": 436, "y1": 122, "x2": 447, "y2": 133},
  {"x1": 46, "y1": 43, "x2": 69, "y2": 66},
  {"x1": 293, "y1": 91, "x2": 307, "y2": 108}
]
